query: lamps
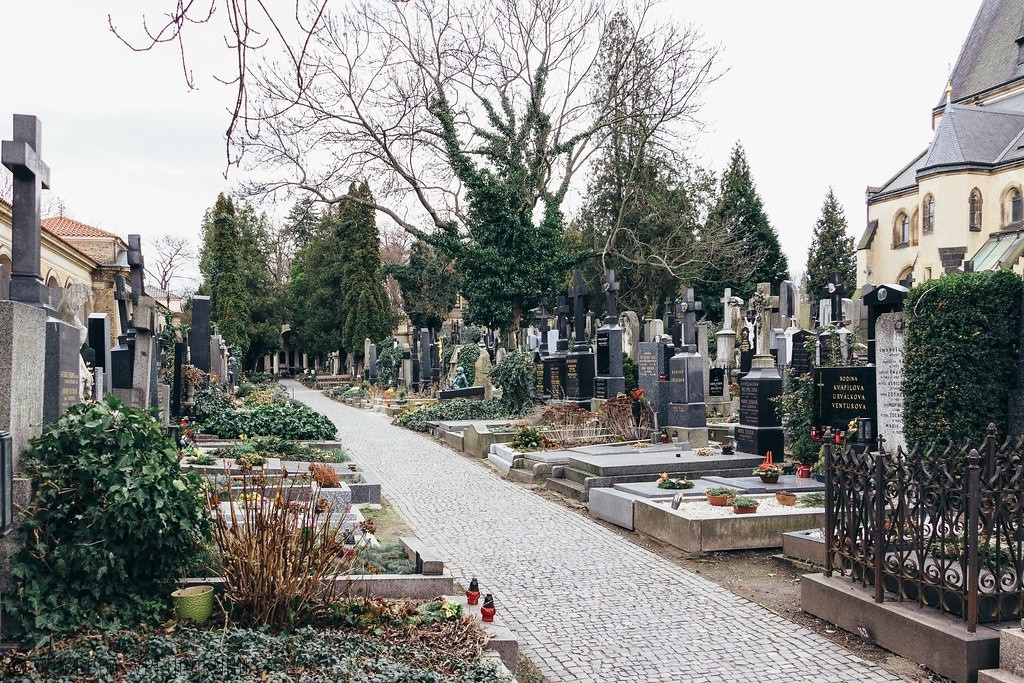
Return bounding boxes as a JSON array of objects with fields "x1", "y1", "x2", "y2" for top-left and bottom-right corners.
[{"x1": 857, "y1": 418, "x2": 873, "y2": 443}]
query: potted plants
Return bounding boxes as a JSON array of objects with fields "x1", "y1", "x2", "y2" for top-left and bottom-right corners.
[
  {"x1": 706, "y1": 487, "x2": 737, "y2": 507},
  {"x1": 791, "y1": 438, "x2": 816, "y2": 478},
  {"x1": 730, "y1": 497, "x2": 759, "y2": 514},
  {"x1": 812, "y1": 458, "x2": 826, "y2": 481}
]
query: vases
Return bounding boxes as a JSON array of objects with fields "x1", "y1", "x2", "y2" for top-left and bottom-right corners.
[
  {"x1": 761, "y1": 475, "x2": 779, "y2": 483},
  {"x1": 171, "y1": 585, "x2": 213, "y2": 623}
]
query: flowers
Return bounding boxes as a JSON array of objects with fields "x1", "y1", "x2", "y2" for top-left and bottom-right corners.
[{"x1": 753, "y1": 466, "x2": 783, "y2": 479}]
